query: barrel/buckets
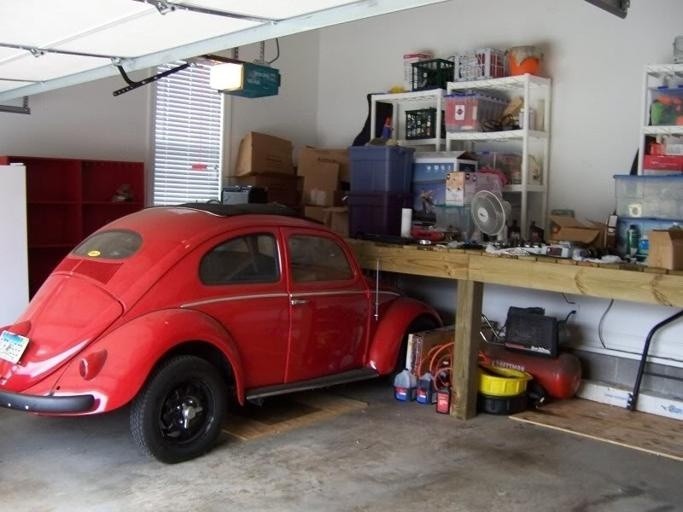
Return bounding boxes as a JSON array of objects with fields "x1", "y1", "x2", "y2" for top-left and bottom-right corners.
[
  {"x1": 393, "y1": 368, "x2": 450, "y2": 414},
  {"x1": 503, "y1": 45, "x2": 542, "y2": 75}
]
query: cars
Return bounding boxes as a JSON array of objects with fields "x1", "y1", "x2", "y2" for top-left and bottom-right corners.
[{"x1": 1, "y1": 200, "x2": 443, "y2": 463}]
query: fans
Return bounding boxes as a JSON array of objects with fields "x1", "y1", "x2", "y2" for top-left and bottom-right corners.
[{"x1": 470, "y1": 189, "x2": 513, "y2": 248}]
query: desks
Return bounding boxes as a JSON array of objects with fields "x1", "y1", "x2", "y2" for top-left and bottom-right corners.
[{"x1": 290, "y1": 239, "x2": 682, "y2": 421}]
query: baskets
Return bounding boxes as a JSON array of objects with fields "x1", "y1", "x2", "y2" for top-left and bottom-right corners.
[
  {"x1": 405, "y1": 109, "x2": 445, "y2": 139},
  {"x1": 454, "y1": 47, "x2": 506, "y2": 82},
  {"x1": 411, "y1": 58, "x2": 453, "y2": 91}
]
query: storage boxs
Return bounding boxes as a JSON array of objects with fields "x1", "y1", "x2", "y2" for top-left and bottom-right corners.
[
  {"x1": 649, "y1": 86, "x2": 683, "y2": 126},
  {"x1": 235, "y1": 131, "x2": 350, "y2": 238},
  {"x1": 549, "y1": 175, "x2": 683, "y2": 270},
  {"x1": 349, "y1": 192, "x2": 413, "y2": 242},
  {"x1": 444, "y1": 91, "x2": 507, "y2": 132},
  {"x1": 411, "y1": 151, "x2": 478, "y2": 224},
  {"x1": 348, "y1": 145, "x2": 416, "y2": 192}
]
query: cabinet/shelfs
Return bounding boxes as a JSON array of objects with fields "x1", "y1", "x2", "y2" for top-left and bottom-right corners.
[
  {"x1": 370, "y1": 87, "x2": 447, "y2": 151},
  {"x1": 445, "y1": 73, "x2": 552, "y2": 242},
  {"x1": 637, "y1": 64, "x2": 683, "y2": 175},
  {"x1": 0, "y1": 155, "x2": 145, "y2": 301}
]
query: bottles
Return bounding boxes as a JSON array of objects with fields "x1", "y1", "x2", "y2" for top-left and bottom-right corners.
[
  {"x1": 628, "y1": 225, "x2": 638, "y2": 255},
  {"x1": 518, "y1": 106, "x2": 535, "y2": 131}
]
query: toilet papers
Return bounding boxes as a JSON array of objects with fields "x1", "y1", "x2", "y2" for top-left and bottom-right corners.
[{"x1": 401, "y1": 208, "x2": 413, "y2": 238}]
